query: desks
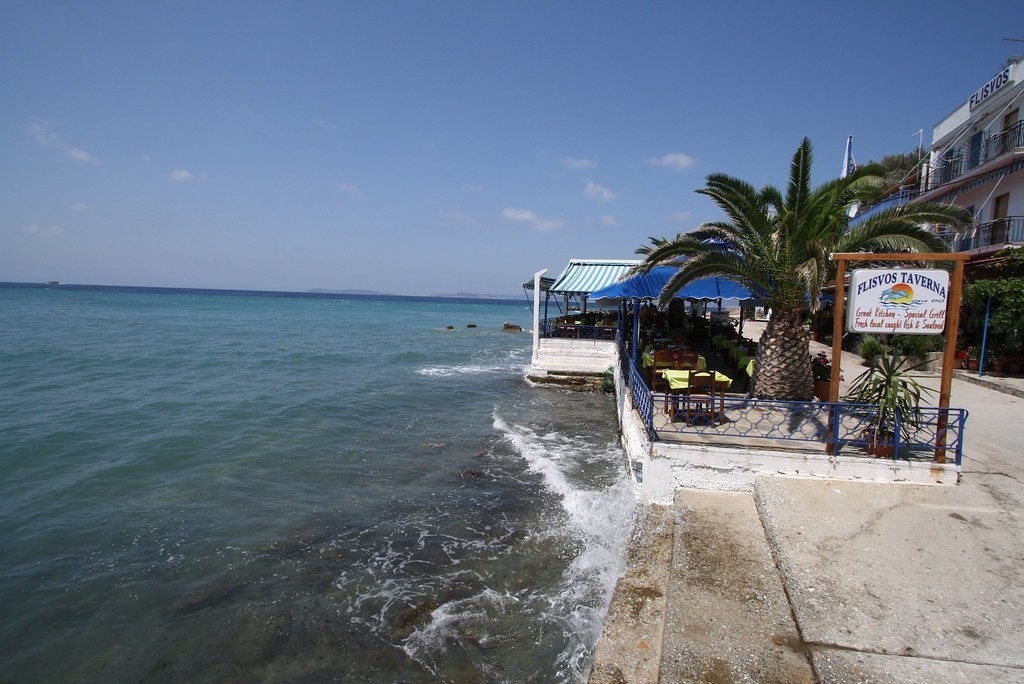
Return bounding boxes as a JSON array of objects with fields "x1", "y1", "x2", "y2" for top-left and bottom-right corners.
[
  {"x1": 630, "y1": 318, "x2": 757, "y2": 422},
  {"x1": 554, "y1": 315, "x2": 619, "y2": 340}
]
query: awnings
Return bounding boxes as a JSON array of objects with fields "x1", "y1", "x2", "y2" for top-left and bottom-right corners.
[{"x1": 523, "y1": 235, "x2": 834, "y2": 367}]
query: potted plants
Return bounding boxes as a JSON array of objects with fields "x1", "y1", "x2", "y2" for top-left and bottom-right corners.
[
  {"x1": 965, "y1": 346, "x2": 1024, "y2": 374},
  {"x1": 844, "y1": 339, "x2": 944, "y2": 457},
  {"x1": 809, "y1": 350, "x2": 844, "y2": 402}
]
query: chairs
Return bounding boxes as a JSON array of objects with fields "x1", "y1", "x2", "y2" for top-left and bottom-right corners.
[
  {"x1": 565, "y1": 316, "x2": 576, "y2": 338},
  {"x1": 602, "y1": 318, "x2": 614, "y2": 340},
  {"x1": 646, "y1": 349, "x2": 676, "y2": 394},
  {"x1": 676, "y1": 350, "x2": 699, "y2": 370},
  {"x1": 685, "y1": 368, "x2": 716, "y2": 427}
]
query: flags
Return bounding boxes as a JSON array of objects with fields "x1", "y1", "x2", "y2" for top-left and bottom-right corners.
[{"x1": 839, "y1": 137, "x2": 857, "y2": 181}]
